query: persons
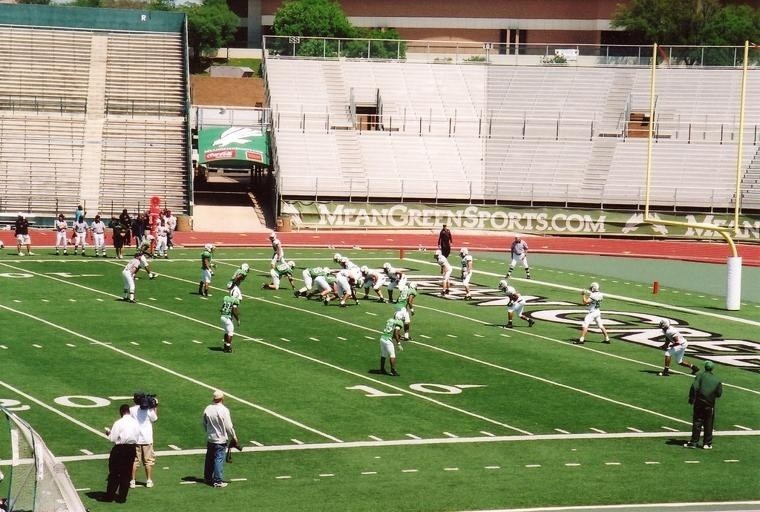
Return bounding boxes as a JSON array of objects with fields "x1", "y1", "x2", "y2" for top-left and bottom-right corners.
[
  {"x1": 433, "y1": 249, "x2": 453, "y2": 296},
  {"x1": 574, "y1": 281, "x2": 610, "y2": 345},
  {"x1": 226, "y1": 263, "x2": 250, "y2": 285},
  {"x1": 504, "y1": 233, "x2": 531, "y2": 279},
  {"x1": 96, "y1": 403, "x2": 139, "y2": 504},
  {"x1": 54, "y1": 205, "x2": 177, "y2": 259},
  {"x1": 134, "y1": 234, "x2": 158, "y2": 279},
  {"x1": 499, "y1": 279, "x2": 535, "y2": 328},
  {"x1": 262, "y1": 260, "x2": 297, "y2": 291},
  {"x1": 219, "y1": 295, "x2": 241, "y2": 353},
  {"x1": 292, "y1": 250, "x2": 418, "y2": 377},
  {"x1": 127, "y1": 391, "x2": 159, "y2": 489},
  {"x1": 269, "y1": 233, "x2": 285, "y2": 266},
  {"x1": 202, "y1": 389, "x2": 238, "y2": 488},
  {"x1": 438, "y1": 224, "x2": 453, "y2": 258},
  {"x1": 459, "y1": 247, "x2": 473, "y2": 298},
  {"x1": 226, "y1": 281, "x2": 243, "y2": 326},
  {"x1": 682, "y1": 360, "x2": 722, "y2": 450},
  {"x1": 13, "y1": 213, "x2": 35, "y2": 256},
  {"x1": 197, "y1": 244, "x2": 212, "y2": 297},
  {"x1": 119, "y1": 253, "x2": 143, "y2": 303},
  {"x1": 657, "y1": 318, "x2": 699, "y2": 376}
]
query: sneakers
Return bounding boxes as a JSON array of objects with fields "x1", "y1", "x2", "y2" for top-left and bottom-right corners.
[
  {"x1": 18, "y1": 251, "x2": 35, "y2": 256},
  {"x1": 573, "y1": 340, "x2": 583, "y2": 344},
  {"x1": 130, "y1": 480, "x2": 154, "y2": 488},
  {"x1": 658, "y1": 371, "x2": 668, "y2": 375},
  {"x1": 684, "y1": 442, "x2": 712, "y2": 449},
  {"x1": 692, "y1": 366, "x2": 699, "y2": 373},
  {"x1": 601, "y1": 340, "x2": 610, "y2": 343},
  {"x1": 214, "y1": 481, "x2": 228, "y2": 487},
  {"x1": 505, "y1": 320, "x2": 534, "y2": 327}
]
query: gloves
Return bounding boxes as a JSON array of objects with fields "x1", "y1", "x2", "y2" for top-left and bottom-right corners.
[{"x1": 397, "y1": 342, "x2": 403, "y2": 352}]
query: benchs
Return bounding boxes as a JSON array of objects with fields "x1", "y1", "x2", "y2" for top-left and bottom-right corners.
[
  {"x1": 264, "y1": 55, "x2": 760, "y2": 209},
  {"x1": 1, "y1": 21, "x2": 190, "y2": 219}
]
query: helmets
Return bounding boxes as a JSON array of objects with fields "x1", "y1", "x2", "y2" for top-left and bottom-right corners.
[
  {"x1": 459, "y1": 248, "x2": 468, "y2": 257},
  {"x1": 334, "y1": 253, "x2": 391, "y2": 273},
  {"x1": 205, "y1": 244, "x2": 213, "y2": 251},
  {"x1": 230, "y1": 289, "x2": 239, "y2": 297},
  {"x1": 288, "y1": 261, "x2": 295, "y2": 268},
  {"x1": 241, "y1": 263, "x2": 249, "y2": 271},
  {"x1": 270, "y1": 232, "x2": 276, "y2": 240},
  {"x1": 434, "y1": 250, "x2": 442, "y2": 258},
  {"x1": 590, "y1": 282, "x2": 599, "y2": 292},
  {"x1": 408, "y1": 280, "x2": 417, "y2": 289},
  {"x1": 659, "y1": 320, "x2": 669, "y2": 328},
  {"x1": 499, "y1": 280, "x2": 507, "y2": 289},
  {"x1": 394, "y1": 312, "x2": 405, "y2": 320}
]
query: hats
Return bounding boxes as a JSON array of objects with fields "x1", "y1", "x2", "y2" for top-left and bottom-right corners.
[{"x1": 705, "y1": 362, "x2": 713, "y2": 369}]
